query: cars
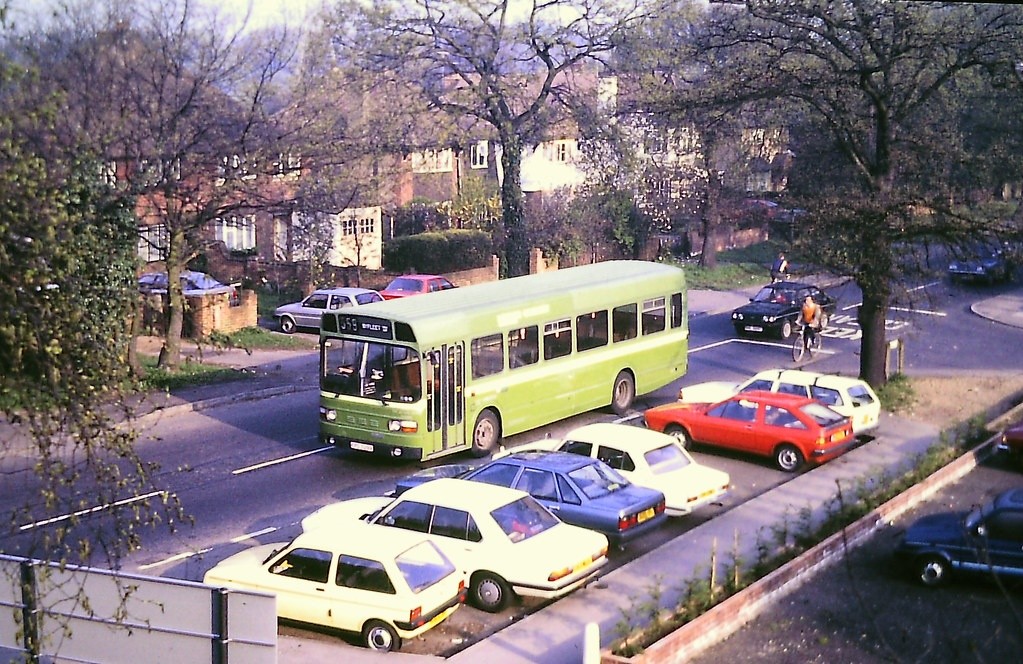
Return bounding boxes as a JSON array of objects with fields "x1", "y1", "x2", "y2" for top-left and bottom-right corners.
[
  {"x1": 730, "y1": 281, "x2": 837, "y2": 339},
  {"x1": 676, "y1": 368, "x2": 882, "y2": 435},
  {"x1": 996, "y1": 417, "x2": 1023, "y2": 472},
  {"x1": 136, "y1": 269, "x2": 240, "y2": 306},
  {"x1": 382, "y1": 449, "x2": 671, "y2": 549},
  {"x1": 893, "y1": 486, "x2": 1023, "y2": 591},
  {"x1": 200, "y1": 522, "x2": 472, "y2": 654},
  {"x1": 743, "y1": 199, "x2": 808, "y2": 227},
  {"x1": 272, "y1": 287, "x2": 386, "y2": 335},
  {"x1": 301, "y1": 477, "x2": 611, "y2": 616},
  {"x1": 785, "y1": 259, "x2": 815, "y2": 276},
  {"x1": 493, "y1": 423, "x2": 731, "y2": 518},
  {"x1": 947, "y1": 243, "x2": 1018, "y2": 287},
  {"x1": 643, "y1": 390, "x2": 856, "y2": 474},
  {"x1": 369, "y1": 274, "x2": 458, "y2": 301}
]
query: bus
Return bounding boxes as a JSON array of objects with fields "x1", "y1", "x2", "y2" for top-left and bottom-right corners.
[{"x1": 317, "y1": 258, "x2": 691, "y2": 463}]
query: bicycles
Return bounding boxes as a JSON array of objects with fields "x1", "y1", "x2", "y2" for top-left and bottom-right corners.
[{"x1": 791, "y1": 321, "x2": 823, "y2": 362}]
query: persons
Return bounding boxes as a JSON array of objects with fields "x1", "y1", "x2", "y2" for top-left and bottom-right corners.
[
  {"x1": 795, "y1": 295, "x2": 821, "y2": 352},
  {"x1": 769, "y1": 252, "x2": 791, "y2": 283}
]
